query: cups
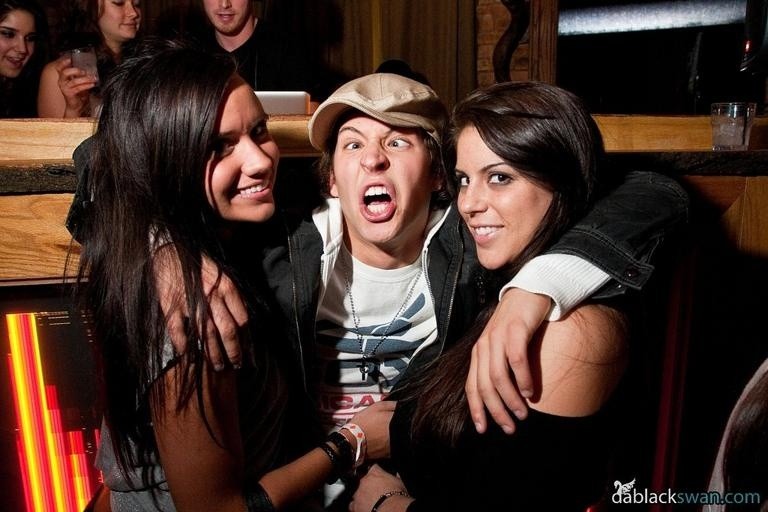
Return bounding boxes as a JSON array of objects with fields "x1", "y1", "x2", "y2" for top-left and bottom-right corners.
[
  {"x1": 710, "y1": 103, "x2": 755, "y2": 152},
  {"x1": 72, "y1": 45, "x2": 100, "y2": 92}
]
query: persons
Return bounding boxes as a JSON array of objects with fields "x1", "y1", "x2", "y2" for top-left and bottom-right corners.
[{"x1": 1, "y1": 0, "x2": 696, "y2": 512}]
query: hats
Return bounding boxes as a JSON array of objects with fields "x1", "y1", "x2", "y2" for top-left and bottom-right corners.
[{"x1": 307, "y1": 71, "x2": 451, "y2": 155}]
